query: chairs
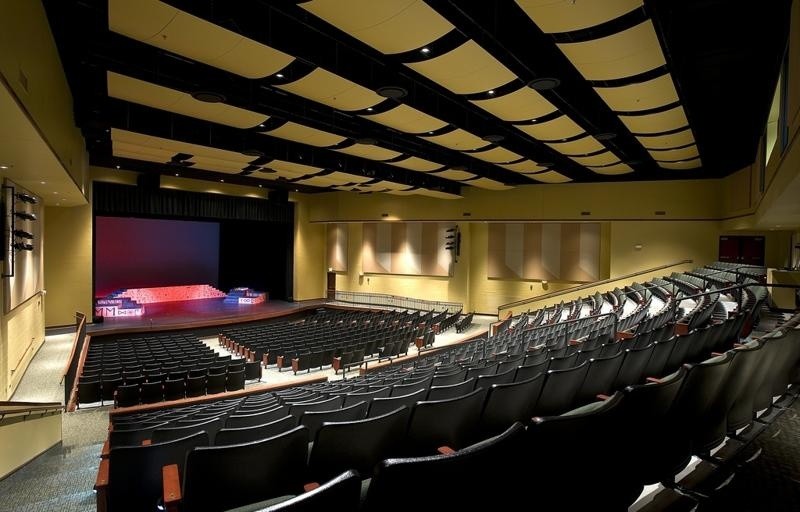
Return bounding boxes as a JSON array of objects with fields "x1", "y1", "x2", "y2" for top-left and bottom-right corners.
[
  {"x1": 109, "y1": 258, "x2": 800, "y2": 511},
  {"x1": 75, "y1": 307, "x2": 475, "y2": 411}
]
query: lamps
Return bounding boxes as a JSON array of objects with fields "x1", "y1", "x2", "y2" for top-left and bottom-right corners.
[
  {"x1": 0, "y1": 183, "x2": 38, "y2": 280},
  {"x1": 444, "y1": 223, "x2": 461, "y2": 262}
]
